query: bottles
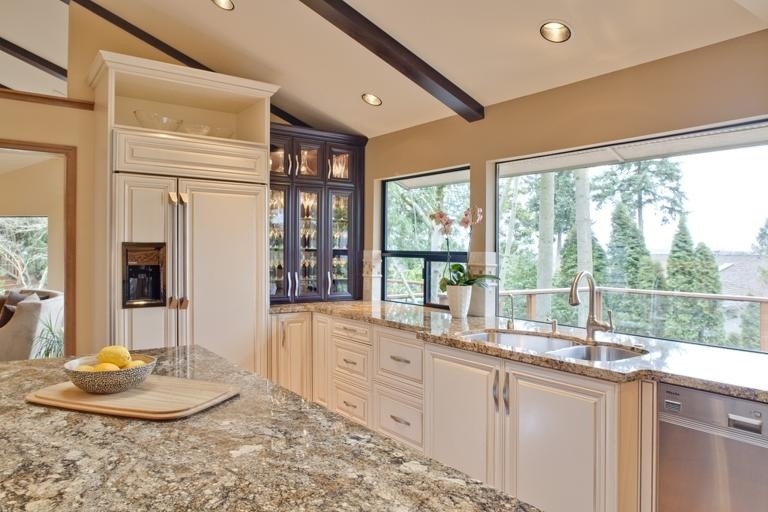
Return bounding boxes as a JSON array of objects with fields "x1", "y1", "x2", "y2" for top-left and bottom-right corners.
[{"x1": 270, "y1": 191, "x2": 348, "y2": 293}]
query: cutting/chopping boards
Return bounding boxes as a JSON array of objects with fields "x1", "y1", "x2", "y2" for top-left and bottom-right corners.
[{"x1": 23, "y1": 374, "x2": 243, "y2": 420}]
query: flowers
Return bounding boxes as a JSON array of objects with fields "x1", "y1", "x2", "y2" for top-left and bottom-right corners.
[{"x1": 425, "y1": 202, "x2": 501, "y2": 293}]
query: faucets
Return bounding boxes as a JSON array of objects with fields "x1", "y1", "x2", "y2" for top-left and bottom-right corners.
[
  {"x1": 568, "y1": 271, "x2": 615, "y2": 340},
  {"x1": 498, "y1": 293, "x2": 515, "y2": 329}
]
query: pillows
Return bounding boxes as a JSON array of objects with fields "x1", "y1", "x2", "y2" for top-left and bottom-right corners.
[
  {"x1": 3, "y1": 287, "x2": 23, "y2": 302},
  {"x1": 17, "y1": 291, "x2": 40, "y2": 304},
  {"x1": 0, "y1": 303, "x2": 13, "y2": 326}
]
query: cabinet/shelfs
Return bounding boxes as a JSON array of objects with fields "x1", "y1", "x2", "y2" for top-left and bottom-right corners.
[
  {"x1": 329, "y1": 320, "x2": 422, "y2": 447},
  {"x1": 90, "y1": 172, "x2": 264, "y2": 372},
  {"x1": 93, "y1": 52, "x2": 272, "y2": 180},
  {"x1": 428, "y1": 351, "x2": 635, "y2": 512},
  {"x1": 274, "y1": 308, "x2": 310, "y2": 396},
  {"x1": 271, "y1": 120, "x2": 366, "y2": 303},
  {"x1": 309, "y1": 312, "x2": 330, "y2": 407}
]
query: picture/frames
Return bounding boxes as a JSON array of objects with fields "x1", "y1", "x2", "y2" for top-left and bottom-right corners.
[{"x1": 121, "y1": 241, "x2": 167, "y2": 309}]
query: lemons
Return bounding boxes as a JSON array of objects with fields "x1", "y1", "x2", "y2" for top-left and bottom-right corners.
[{"x1": 76, "y1": 345, "x2": 145, "y2": 372}]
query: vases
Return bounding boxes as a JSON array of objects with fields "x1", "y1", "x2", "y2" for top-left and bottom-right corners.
[{"x1": 445, "y1": 284, "x2": 472, "y2": 316}]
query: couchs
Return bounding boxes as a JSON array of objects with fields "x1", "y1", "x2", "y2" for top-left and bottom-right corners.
[{"x1": 0, "y1": 287, "x2": 64, "y2": 360}]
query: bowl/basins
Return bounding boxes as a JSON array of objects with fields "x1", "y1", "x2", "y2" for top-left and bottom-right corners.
[{"x1": 62, "y1": 352, "x2": 157, "y2": 393}]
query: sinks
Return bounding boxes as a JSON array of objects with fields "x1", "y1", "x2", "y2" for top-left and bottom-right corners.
[
  {"x1": 461, "y1": 332, "x2": 582, "y2": 353},
  {"x1": 547, "y1": 345, "x2": 649, "y2": 362}
]
